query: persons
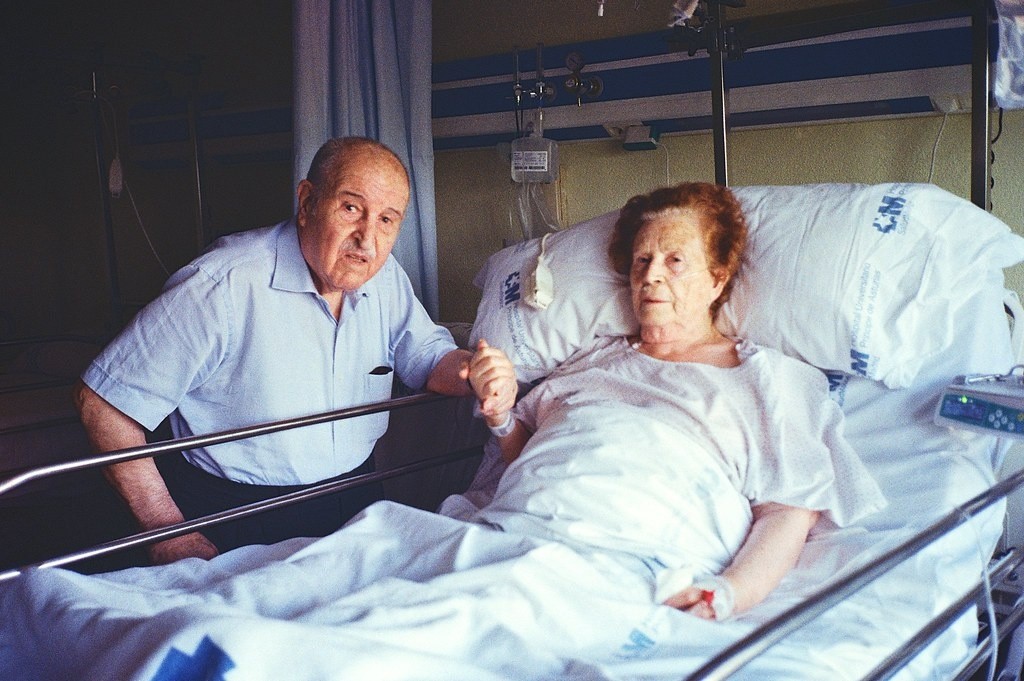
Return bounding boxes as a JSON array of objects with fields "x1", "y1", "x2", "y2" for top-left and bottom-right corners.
[
  {"x1": 25, "y1": 183, "x2": 839, "y2": 681},
  {"x1": 73, "y1": 135, "x2": 518, "y2": 567}
]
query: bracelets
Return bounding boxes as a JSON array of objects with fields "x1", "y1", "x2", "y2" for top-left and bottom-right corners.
[{"x1": 486, "y1": 411, "x2": 514, "y2": 437}]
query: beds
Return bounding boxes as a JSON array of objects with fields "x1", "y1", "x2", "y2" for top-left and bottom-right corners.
[{"x1": 0, "y1": 371, "x2": 1024, "y2": 681}]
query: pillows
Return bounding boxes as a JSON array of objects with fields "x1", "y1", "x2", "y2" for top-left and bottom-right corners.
[{"x1": 469, "y1": 178, "x2": 1023, "y2": 390}]
